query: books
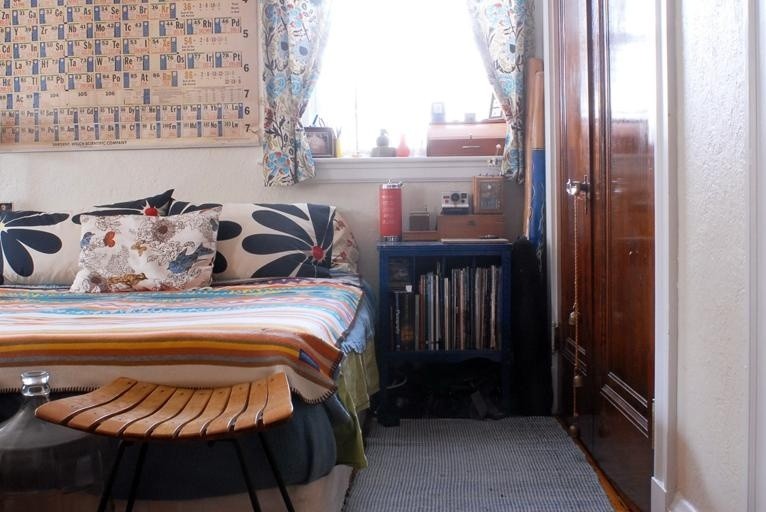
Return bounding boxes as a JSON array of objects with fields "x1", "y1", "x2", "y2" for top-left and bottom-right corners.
[
  {"x1": 390, "y1": 264, "x2": 503, "y2": 351},
  {"x1": 441, "y1": 238, "x2": 509, "y2": 244}
]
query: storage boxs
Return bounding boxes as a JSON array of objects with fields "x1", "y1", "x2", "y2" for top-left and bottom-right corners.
[{"x1": 426, "y1": 120, "x2": 511, "y2": 156}]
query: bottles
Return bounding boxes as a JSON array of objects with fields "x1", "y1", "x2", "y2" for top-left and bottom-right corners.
[{"x1": 380, "y1": 183, "x2": 403, "y2": 243}]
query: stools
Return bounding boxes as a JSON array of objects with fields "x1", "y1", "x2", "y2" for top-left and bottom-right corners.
[{"x1": 33, "y1": 373, "x2": 295, "y2": 512}]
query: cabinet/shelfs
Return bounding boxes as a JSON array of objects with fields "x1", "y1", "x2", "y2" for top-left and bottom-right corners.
[
  {"x1": 557, "y1": 0, "x2": 655, "y2": 512},
  {"x1": 378, "y1": 241, "x2": 513, "y2": 402}
]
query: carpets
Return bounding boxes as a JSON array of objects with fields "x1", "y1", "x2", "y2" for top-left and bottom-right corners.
[{"x1": 342, "y1": 415, "x2": 615, "y2": 511}]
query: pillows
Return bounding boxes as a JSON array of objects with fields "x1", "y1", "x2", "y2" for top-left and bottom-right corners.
[
  {"x1": 1, "y1": 186, "x2": 174, "y2": 290},
  {"x1": 69, "y1": 206, "x2": 223, "y2": 294},
  {"x1": 168, "y1": 202, "x2": 359, "y2": 280}
]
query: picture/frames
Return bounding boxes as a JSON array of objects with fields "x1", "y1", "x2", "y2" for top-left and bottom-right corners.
[
  {"x1": 475, "y1": 176, "x2": 505, "y2": 214},
  {"x1": 301, "y1": 126, "x2": 334, "y2": 157}
]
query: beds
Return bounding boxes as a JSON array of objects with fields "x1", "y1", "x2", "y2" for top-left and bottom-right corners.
[{"x1": 1, "y1": 187, "x2": 380, "y2": 500}]
